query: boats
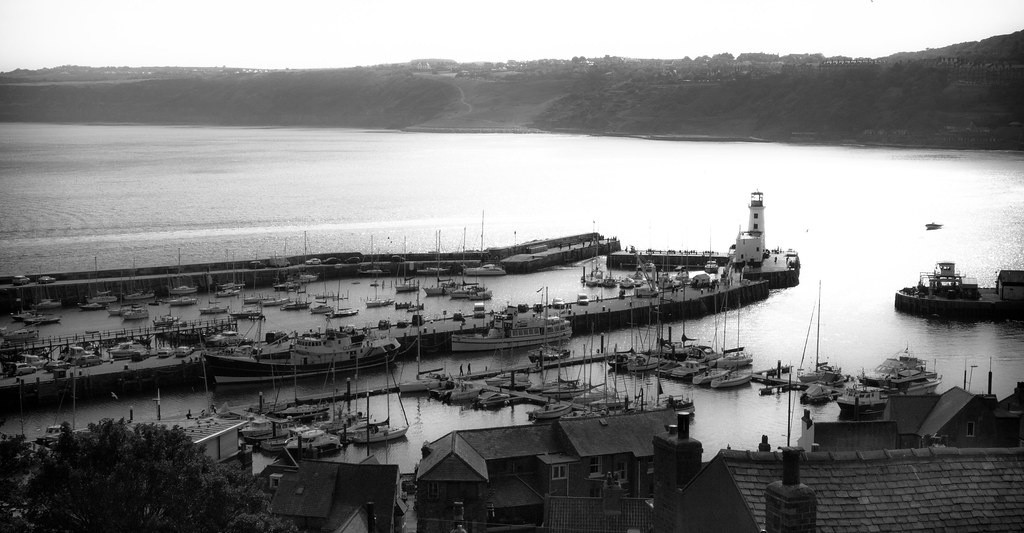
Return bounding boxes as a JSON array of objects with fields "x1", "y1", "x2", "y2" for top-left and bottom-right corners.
[
  {"x1": 1, "y1": 230, "x2": 1021, "y2": 461},
  {"x1": 925, "y1": 223, "x2": 943, "y2": 230}
]
